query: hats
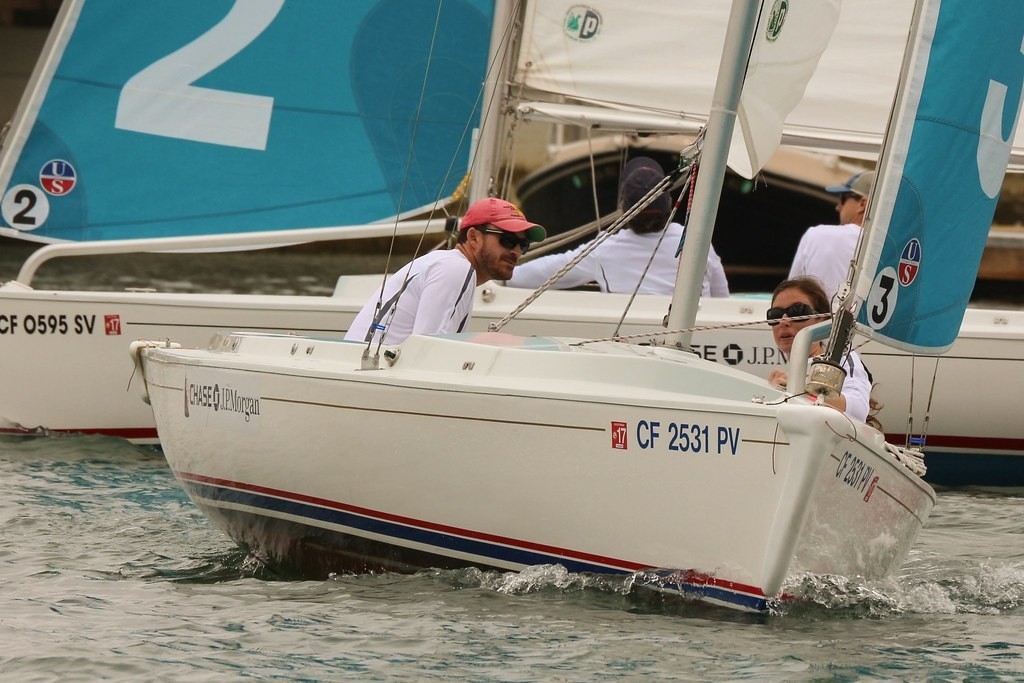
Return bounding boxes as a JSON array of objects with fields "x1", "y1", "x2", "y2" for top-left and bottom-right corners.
[
  {"x1": 824, "y1": 170, "x2": 875, "y2": 198},
  {"x1": 621, "y1": 157, "x2": 670, "y2": 235},
  {"x1": 460, "y1": 198, "x2": 546, "y2": 242}
]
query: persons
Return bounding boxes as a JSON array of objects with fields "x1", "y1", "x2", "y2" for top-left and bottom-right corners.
[
  {"x1": 768, "y1": 278, "x2": 873, "y2": 423},
  {"x1": 344, "y1": 198, "x2": 546, "y2": 345},
  {"x1": 495, "y1": 157, "x2": 729, "y2": 296},
  {"x1": 787, "y1": 170, "x2": 874, "y2": 311}
]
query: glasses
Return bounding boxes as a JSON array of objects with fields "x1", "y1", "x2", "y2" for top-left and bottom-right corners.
[
  {"x1": 839, "y1": 193, "x2": 862, "y2": 205},
  {"x1": 477, "y1": 228, "x2": 530, "y2": 255},
  {"x1": 767, "y1": 304, "x2": 818, "y2": 326}
]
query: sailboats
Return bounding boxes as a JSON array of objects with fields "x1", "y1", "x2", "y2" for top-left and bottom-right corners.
[{"x1": 1, "y1": 0, "x2": 1024, "y2": 620}]
